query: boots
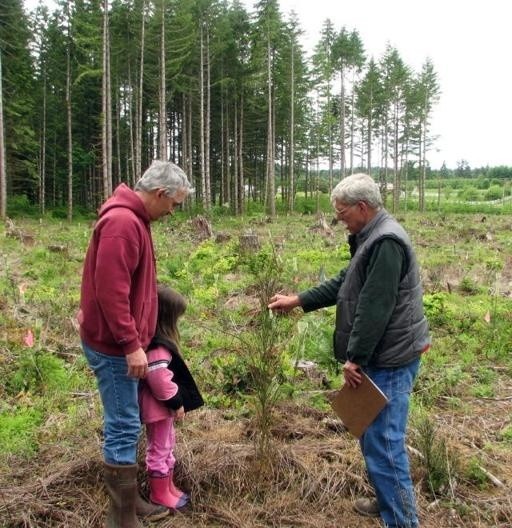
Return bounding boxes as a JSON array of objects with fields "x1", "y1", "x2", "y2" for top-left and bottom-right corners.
[
  {"x1": 103, "y1": 461, "x2": 170, "y2": 528},
  {"x1": 149, "y1": 468, "x2": 189, "y2": 508}
]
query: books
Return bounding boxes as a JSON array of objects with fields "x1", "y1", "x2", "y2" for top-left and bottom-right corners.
[{"x1": 331, "y1": 371, "x2": 391, "y2": 441}]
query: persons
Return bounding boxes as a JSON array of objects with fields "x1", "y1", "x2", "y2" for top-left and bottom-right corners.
[
  {"x1": 78, "y1": 157, "x2": 192, "y2": 526},
  {"x1": 143, "y1": 283, "x2": 206, "y2": 508},
  {"x1": 268, "y1": 172, "x2": 432, "y2": 528}
]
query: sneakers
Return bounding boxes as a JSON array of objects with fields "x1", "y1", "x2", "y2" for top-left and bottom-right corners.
[{"x1": 353, "y1": 497, "x2": 381, "y2": 517}]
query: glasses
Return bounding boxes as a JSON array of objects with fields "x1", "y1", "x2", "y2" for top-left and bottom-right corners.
[{"x1": 335, "y1": 204, "x2": 352, "y2": 216}]
